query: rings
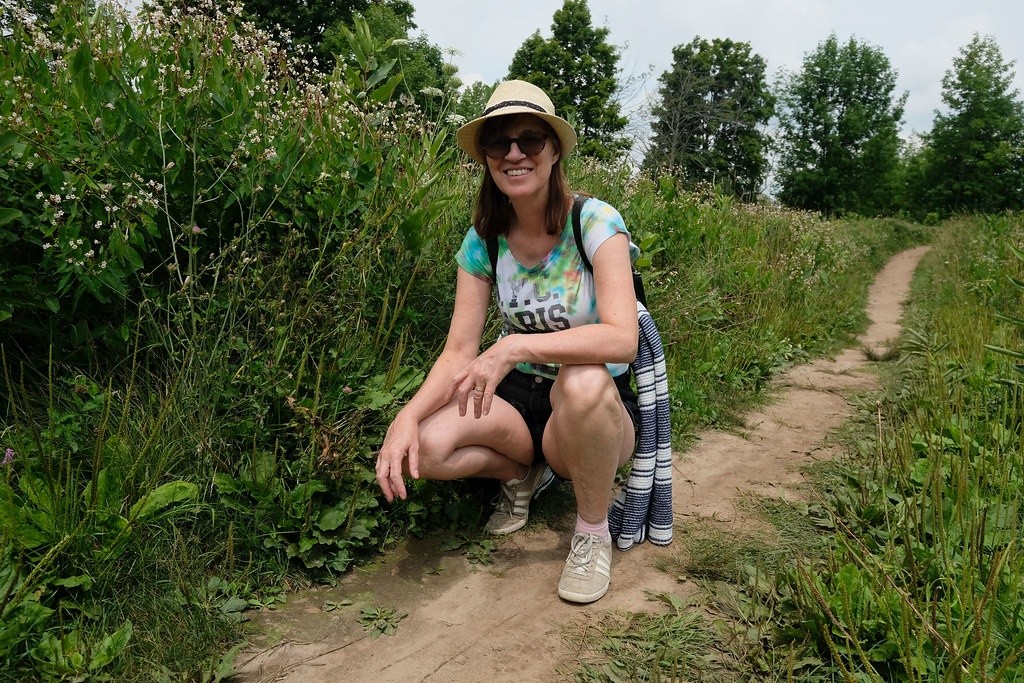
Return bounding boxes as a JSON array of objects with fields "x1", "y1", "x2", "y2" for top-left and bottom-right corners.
[{"x1": 475, "y1": 385, "x2": 484, "y2": 392}]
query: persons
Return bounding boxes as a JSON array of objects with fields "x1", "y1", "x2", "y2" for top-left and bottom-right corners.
[{"x1": 375, "y1": 80, "x2": 641, "y2": 603}]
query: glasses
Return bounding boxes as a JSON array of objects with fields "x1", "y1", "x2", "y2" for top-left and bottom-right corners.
[{"x1": 483, "y1": 131, "x2": 550, "y2": 160}]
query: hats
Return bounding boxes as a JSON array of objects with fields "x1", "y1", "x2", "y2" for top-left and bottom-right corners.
[{"x1": 455, "y1": 80, "x2": 578, "y2": 168}]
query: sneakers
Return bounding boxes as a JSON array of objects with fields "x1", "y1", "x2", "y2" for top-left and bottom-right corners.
[
  {"x1": 485, "y1": 461, "x2": 555, "y2": 535},
  {"x1": 558, "y1": 532, "x2": 612, "y2": 603}
]
